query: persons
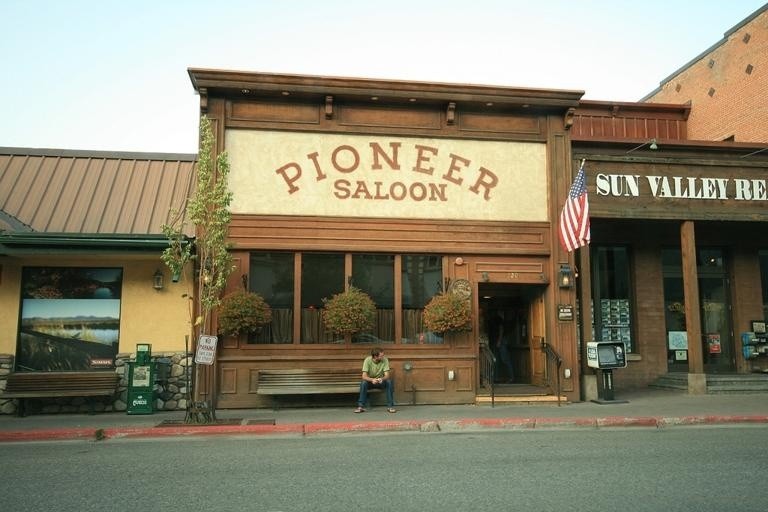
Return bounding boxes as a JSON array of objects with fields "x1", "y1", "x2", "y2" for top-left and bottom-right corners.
[
  {"x1": 487, "y1": 308, "x2": 514, "y2": 383},
  {"x1": 354, "y1": 348, "x2": 395, "y2": 413}
]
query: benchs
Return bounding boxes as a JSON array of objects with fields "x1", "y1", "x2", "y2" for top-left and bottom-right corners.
[
  {"x1": 256, "y1": 369, "x2": 398, "y2": 401},
  {"x1": 0, "y1": 373, "x2": 119, "y2": 416}
]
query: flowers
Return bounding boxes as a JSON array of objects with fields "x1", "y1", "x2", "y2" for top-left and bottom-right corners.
[
  {"x1": 323, "y1": 288, "x2": 376, "y2": 334},
  {"x1": 423, "y1": 294, "x2": 471, "y2": 336},
  {"x1": 215, "y1": 291, "x2": 273, "y2": 334}
]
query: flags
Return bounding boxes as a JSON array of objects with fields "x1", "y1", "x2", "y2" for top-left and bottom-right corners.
[{"x1": 557, "y1": 166, "x2": 590, "y2": 253}]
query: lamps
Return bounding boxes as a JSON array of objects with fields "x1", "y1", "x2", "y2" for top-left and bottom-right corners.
[
  {"x1": 626, "y1": 136, "x2": 658, "y2": 154},
  {"x1": 150, "y1": 268, "x2": 168, "y2": 291},
  {"x1": 558, "y1": 265, "x2": 575, "y2": 288}
]
